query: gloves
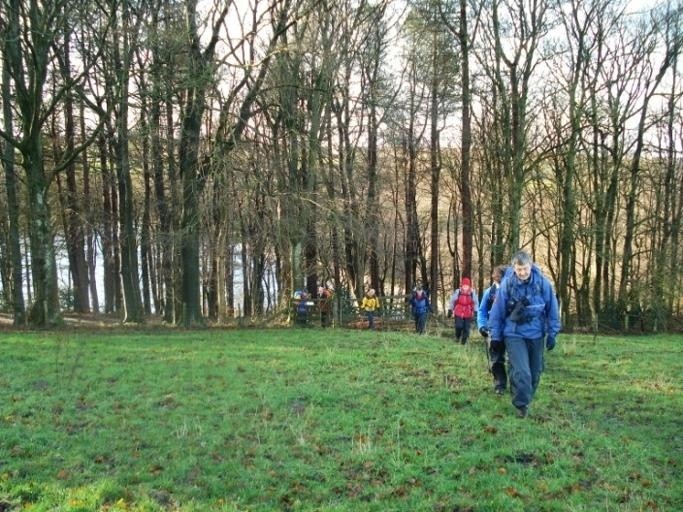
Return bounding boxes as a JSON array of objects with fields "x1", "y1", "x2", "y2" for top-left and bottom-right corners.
[
  {"x1": 481, "y1": 327, "x2": 488, "y2": 337},
  {"x1": 546, "y1": 335, "x2": 555, "y2": 350},
  {"x1": 448, "y1": 310, "x2": 452, "y2": 318}
]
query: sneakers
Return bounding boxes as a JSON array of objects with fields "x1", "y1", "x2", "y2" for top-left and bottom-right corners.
[
  {"x1": 496, "y1": 389, "x2": 504, "y2": 396},
  {"x1": 455, "y1": 337, "x2": 459, "y2": 342},
  {"x1": 518, "y1": 405, "x2": 527, "y2": 417}
]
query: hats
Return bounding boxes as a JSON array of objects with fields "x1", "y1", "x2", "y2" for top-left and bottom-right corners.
[{"x1": 462, "y1": 278, "x2": 470, "y2": 285}]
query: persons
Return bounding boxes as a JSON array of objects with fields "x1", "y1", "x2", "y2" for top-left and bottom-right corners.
[
  {"x1": 359, "y1": 288, "x2": 381, "y2": 329},
  {"x1": 447, "y1": 276, "x2": 479, "y2": 347},
  {"x1": 488, "y1": 252, "x2": 559, "y2": 420},
  {"x1": 407, "y1": 278, "x2": 434, "y2": 334},
  {"x1": 317, "y1": 286, "x2": 334, "y2": 328},
  {"x1": 476, "y1": 265, "x2": 509, "y2": 396},
  {"x1": 294, "y1": 288, "x2": 310, "y2": 325}
]
